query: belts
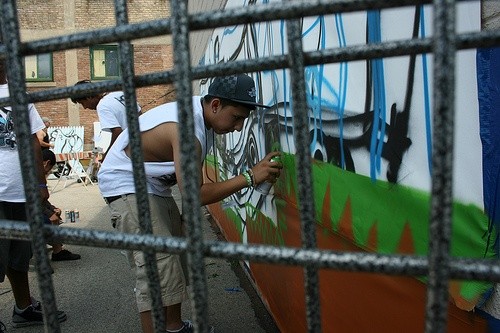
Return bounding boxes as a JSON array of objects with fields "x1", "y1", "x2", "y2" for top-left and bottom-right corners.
[{"x1": 105, "y1": 193, "x2": 134, "y2": 203}]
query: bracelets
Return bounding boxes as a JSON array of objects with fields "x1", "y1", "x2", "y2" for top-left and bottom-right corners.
[{"x1": 242, "y1": 168, "x2": 255, "y2": 188}]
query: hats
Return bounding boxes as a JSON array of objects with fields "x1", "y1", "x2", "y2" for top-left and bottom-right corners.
[
  {"x1": 42, "y1": 117, "x2": 49, "y2": 122},
  {"x1": 208, "y1": 73, "x2": 272, "y2": 110}
]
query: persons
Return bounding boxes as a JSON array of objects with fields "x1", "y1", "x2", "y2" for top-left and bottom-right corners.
[
  {"x1": 41, "y1": 117, "x2": 56, "y2": 152},
  {"x1": 69, "y1": 78, "x2": 143, "y2": 163},
  {"x1": 0, "y1": 15, "x2": 69, "y2": 333},
  {"x1": 98, "y1": 73, "x2": 283, "y2": 332},
  {"x1": 41, "y1": 149, "x2": 82, "y2": 262}
]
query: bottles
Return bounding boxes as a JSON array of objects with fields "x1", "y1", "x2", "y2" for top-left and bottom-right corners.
[
  {"x1": 253, "y1": 154, "x2": 282, "y2": 195},
  {"x1": 65, "y1": 209, "x2": 79, "y2": 222},
  {"x1": 94, "y1": 148, "x2": 98, "y2": 163}
]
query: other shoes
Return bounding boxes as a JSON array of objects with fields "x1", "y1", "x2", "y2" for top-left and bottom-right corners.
[
  {"x1": 165, "y1": 319, "x2": 195, "y2": 333},
  {"x1": 11, "y1": 296, "x2": 67, "y2": 328},
  {"x1": 51, "y1": 249, "x2": 81, "y2": 261}
]
